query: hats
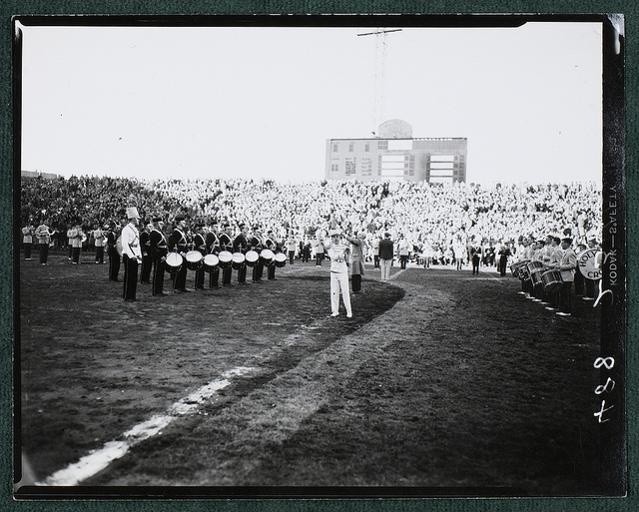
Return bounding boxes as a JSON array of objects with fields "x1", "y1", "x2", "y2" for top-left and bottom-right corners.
[
  {"x1": 126, "y1": 206, "x2": 141, "y2": 220},
  {"x1": 329, "y1": 229, "x2": 341, "y2": 238}
]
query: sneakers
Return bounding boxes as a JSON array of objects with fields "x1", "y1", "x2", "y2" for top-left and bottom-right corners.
[{"x1": 330, "y1": 311, "x2": 354, "y2": 320}]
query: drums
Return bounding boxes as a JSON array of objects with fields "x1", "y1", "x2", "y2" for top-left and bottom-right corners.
[
  {"x1": 511, "y1": 261, "x2": 562, "y2": 293},
  {"x1": 164, "y1": 249, "x2": 287, "y2": 274}
]
